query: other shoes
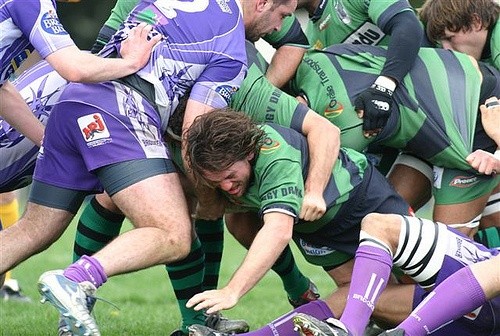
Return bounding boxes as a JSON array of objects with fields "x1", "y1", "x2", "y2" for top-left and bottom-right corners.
[{"x1": 0, "y1": 280, "x2": 30, "y2": 303}]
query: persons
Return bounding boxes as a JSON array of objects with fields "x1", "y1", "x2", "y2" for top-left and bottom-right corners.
[
  {"x1": 0, "y1": 0, "x2": 298, "y2": 336},
  {"x1": 0, "y1": 1, "x2": 500, "y2": 336}
]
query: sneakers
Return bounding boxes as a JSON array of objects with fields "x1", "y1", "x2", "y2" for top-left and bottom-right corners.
[
  {"x1": 37, "y1": 270, "x2": 120, "y2": 336},
  {"x1": 182, "y1": 312, "x2": 249, "y2": 336},
  {"x1": 293, "y1": 313, "x2": 348, "y2": 336},
  {"x1": 288, "y1": 280, "x2": 320, "y2": 308}
]
